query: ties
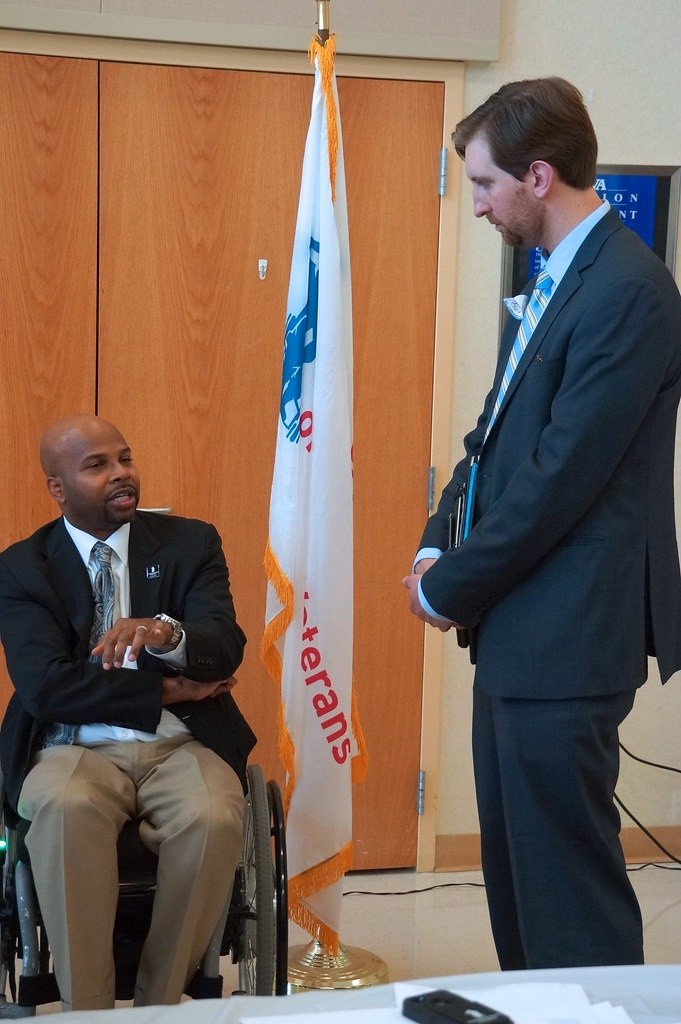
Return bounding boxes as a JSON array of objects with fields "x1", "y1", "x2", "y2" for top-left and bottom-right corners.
[
  {"x1": 38, "y1": 542, "x2": 116, "y2": 751},
  {"x1": 484, "y1": 265, "x2": 555, "y2": 448}
]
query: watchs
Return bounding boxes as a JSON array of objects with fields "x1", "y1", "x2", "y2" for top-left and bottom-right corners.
[{"x1": 153, "y1": 613, "x2": 182, "y2": 654}]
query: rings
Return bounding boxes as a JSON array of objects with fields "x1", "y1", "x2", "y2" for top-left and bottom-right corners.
[{"x1": 135, "y1": 625, "x2": 148, "y2": 633}]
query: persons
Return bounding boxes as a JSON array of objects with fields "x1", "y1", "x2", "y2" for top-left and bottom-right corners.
[
  {"x1": 0, "y1": 414, "x2": 258, "y2": 1010},
  {"x1": 402, "y1": 77, "x2": 681, "y2": 970}
]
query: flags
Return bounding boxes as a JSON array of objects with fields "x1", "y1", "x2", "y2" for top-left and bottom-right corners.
[{"x1": 259, "y1": 30, "x2": 369, "y2": 958}]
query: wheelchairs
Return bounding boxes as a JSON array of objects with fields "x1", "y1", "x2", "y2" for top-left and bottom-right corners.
[{"x1": 0, "y1": 762, "x2": 291, "y2": 1014}]
